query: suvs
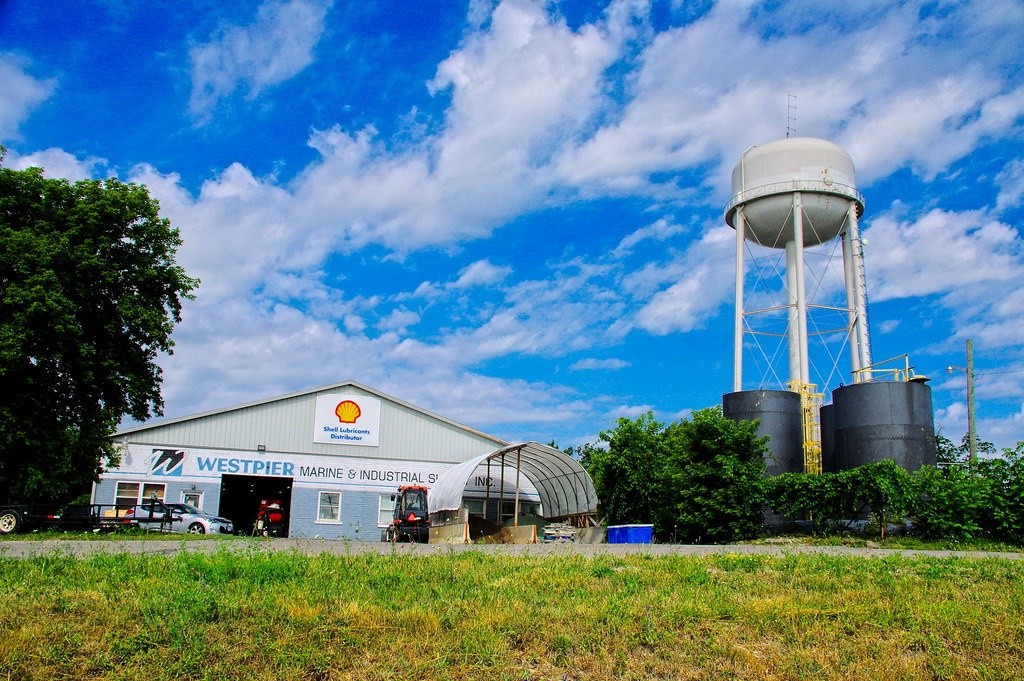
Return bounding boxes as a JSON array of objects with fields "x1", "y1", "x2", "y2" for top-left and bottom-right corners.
[{"x1": 120, "y1": 503, "x2": 233, "y2": 537}]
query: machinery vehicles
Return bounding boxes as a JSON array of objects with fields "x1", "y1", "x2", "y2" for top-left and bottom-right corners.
[{"x1": 381, "y1": 483, "x2": 432, "y2": 544}]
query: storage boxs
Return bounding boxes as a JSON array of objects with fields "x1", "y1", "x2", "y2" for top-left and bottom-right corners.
[
  {"x1": 550, "y1": 535, "x2": 567, "y2": 541},
  {"x1": 607, "y1": 524, "x2": 655, "y2": 544}
]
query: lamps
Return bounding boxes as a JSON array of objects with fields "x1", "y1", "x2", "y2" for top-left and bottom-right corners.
[{"x1": 258, "y1": 445, "x2": 265, "y2": 451}]
